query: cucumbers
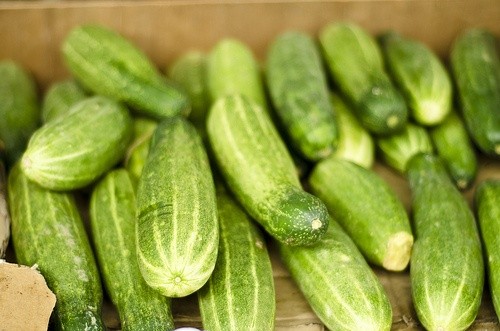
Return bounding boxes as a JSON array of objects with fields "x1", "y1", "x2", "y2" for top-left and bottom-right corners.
[{"x1": 1, "y1": 19, "x2": 500, "y2": 331}]
